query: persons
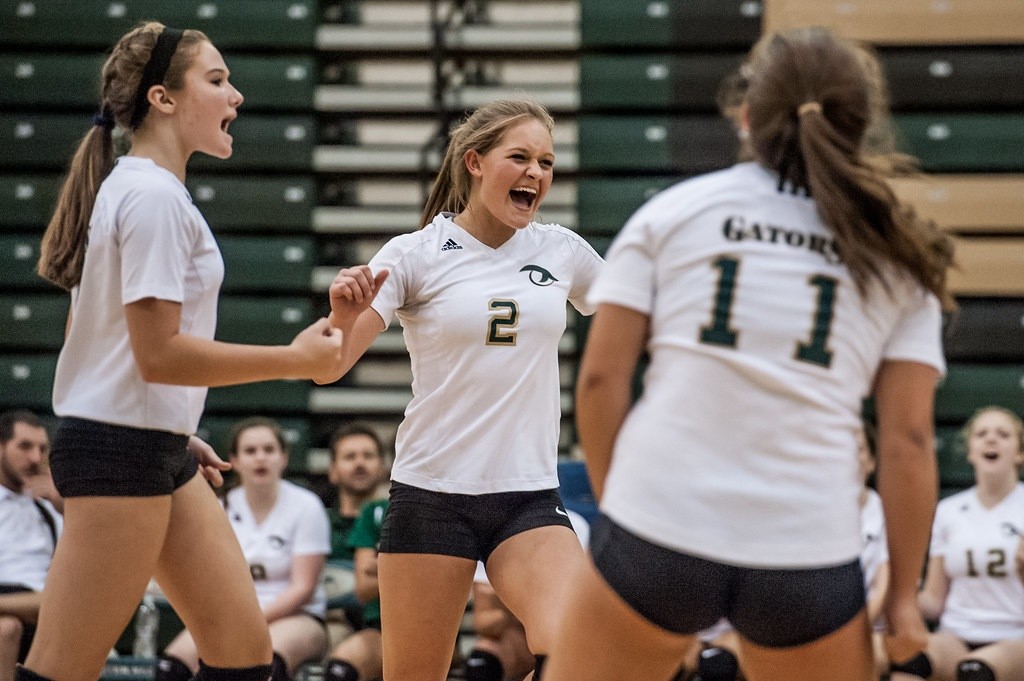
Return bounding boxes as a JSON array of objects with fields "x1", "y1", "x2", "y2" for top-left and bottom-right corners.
[
  {"x1": 0, "y1": 407, "x2": 1024, "y2": 680},
  {"x1": 10, "y1": 19, "x2": 345, "y2": 681},
  {"x1": 540, "y1": 25, "x2": 966, "y2": 681},
  {"x1": 314, "y1": 99, "x2": 609, "y2": 681}
]
